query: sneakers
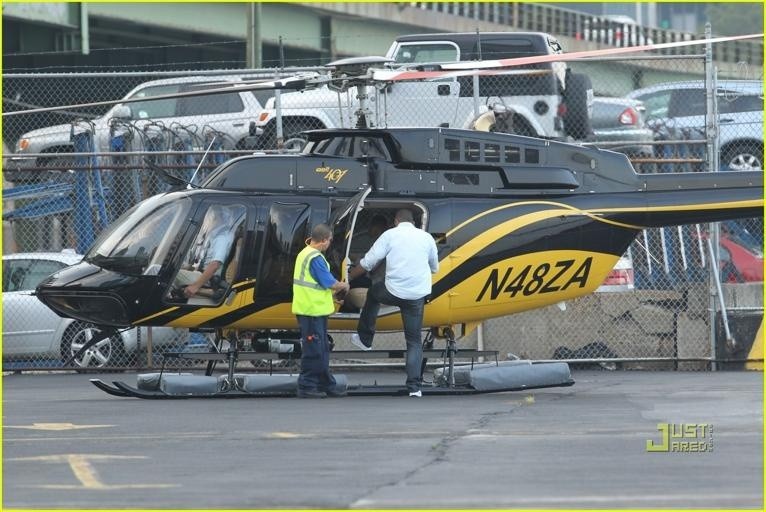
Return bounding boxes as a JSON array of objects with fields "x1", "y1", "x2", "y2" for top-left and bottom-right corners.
[
  {"x1": 296, "y1": 388, "x2": 327, "y2": 398},
  {"x1": 410, "y1": 390, "x2": 421, "y2": 398},
  {"x1": 351, "y1": 334, "x2": 370, "y2": 351},
  {"x1": 325, "y1": 390, "x2": 347, "y2": 398}
]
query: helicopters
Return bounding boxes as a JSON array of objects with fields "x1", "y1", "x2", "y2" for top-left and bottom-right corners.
[{"x1": 2, "y1": 33, "x2": 765, "y2": 398}]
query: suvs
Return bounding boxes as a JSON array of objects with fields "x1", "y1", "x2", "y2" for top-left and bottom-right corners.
[
  {"x1": 5, "y1": 73, "x2": 323, "y2": 181},
  {"x1": 249, "y1": 30, "x2": 595, "y2": 159}
]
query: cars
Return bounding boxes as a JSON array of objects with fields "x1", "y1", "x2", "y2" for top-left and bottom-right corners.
[
  {"x1": 1, "y1": 249, "x2": 191, "y2": 374},
  {"x1": 591, "y1": 96, "x2": 654, "y2": 156},
  {"x1": 577, "y1": 14, "x2": 653, "y2": 47}
]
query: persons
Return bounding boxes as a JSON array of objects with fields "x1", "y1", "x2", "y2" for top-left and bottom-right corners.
[
  {"x1": 336, "y1": 216, "x2": 388, "y2": 312},
  {"x1": 144, "y1": 204, "x2": 235, "y2": 300},
  {"x1": 349, "y1": 208, "x2": 439, "y2": 398},
  {"x1": 291, "y1": 223, "x2": 350, "y2": 399}
]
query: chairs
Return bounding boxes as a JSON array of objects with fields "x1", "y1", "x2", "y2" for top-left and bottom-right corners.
[{"x1": 219, "y1": 226, "x2": 242, "y2": 284}]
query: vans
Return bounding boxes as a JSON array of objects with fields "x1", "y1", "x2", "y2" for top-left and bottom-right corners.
[{"x1": 623, "y1": 79, "x2": 765, "y2": 172}]
